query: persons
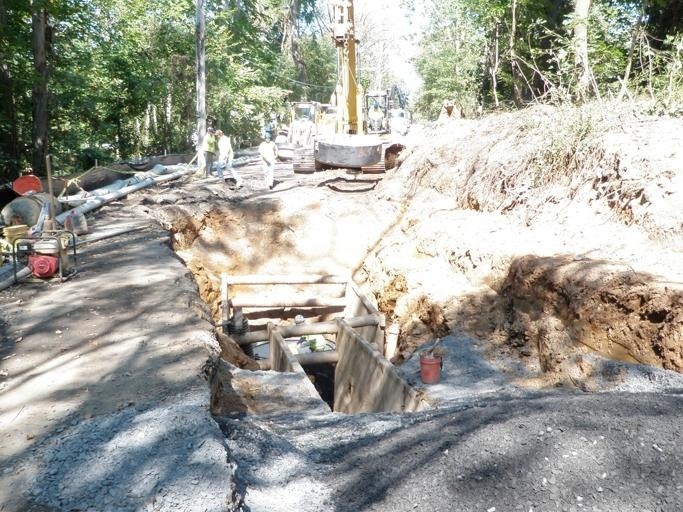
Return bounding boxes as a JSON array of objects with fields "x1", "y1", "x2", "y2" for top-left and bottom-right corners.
[
  {"x1": 367, "y1": 103, "x2": 387, "y2": 130},
  {"x1": 212, "y1": 129, "x2": 236, "y2": 183},
  {"x1": 201, "y1": 127, "x2": 218, "y2": 178},
  {"x1": 257, "y1": 132, "x2": 279, "y2": 190}
]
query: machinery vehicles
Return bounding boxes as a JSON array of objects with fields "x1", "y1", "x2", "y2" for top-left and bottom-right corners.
[{"x1": 272, "y1": 1, "x2": 408, "y2": 177}]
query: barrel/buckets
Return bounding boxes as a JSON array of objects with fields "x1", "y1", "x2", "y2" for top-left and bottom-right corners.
[
  {"x1": 13, "y1": 174, "x2": 43, "y2": 195},
  {"x1": 2, "y1": 224, "x2": 29, "y2": 246},
  {"x1": 418, "y1": 355, "x2": 443, "y2": 384},
  {"x1": 70, "y1": 212, "x2": 89, "y2": 234}
]
